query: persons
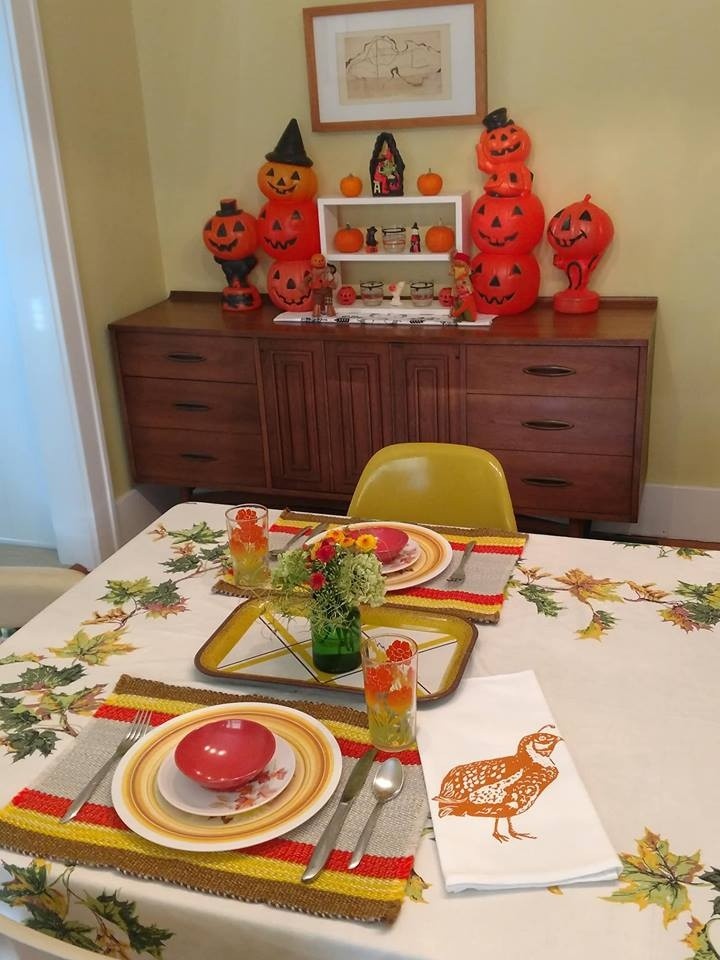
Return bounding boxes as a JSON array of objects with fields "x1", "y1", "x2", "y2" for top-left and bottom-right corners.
[
  {"x1": 409, "y1": 223, "x2": 421, "y2": 254},
  {"x1": 448, "y1": 254, "x2": 477, "y2": 324},
  {"x1": 304, "y1": 254, "x2": 337, "y2": 318}
]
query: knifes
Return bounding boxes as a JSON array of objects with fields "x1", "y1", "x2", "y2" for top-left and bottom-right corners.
[{"x1": 302, "y1": 744, "x2": 377, "y2": 884}]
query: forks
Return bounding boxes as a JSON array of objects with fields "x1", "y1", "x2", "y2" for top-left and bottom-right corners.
[
  {"x1": 446, "y1": 540, "x2": 475, "y2": 583},
  {"x1": 57, "y1": 708, "x2": 155, "y2": 824}
]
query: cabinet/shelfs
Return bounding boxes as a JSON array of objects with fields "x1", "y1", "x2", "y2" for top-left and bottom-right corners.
[
  {"x1": 115, "y1": 331, "x2": 268, "y2": 490},
  {"x1": 465, "y1": 344, "x2": 640, "y2": 519},
  {"x1": 317, "y1": 193, "x2": 471, "y2": 303},
  {"x1": 256, "y1": 337, "x2": 460, "y2": 497}
]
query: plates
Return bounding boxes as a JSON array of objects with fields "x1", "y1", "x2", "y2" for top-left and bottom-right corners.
[
  {"x1": 110, "y1": 702, "x2": 342, "y2": 854},
  {"x1": 159, "y1": 738, "x2": 296, "y2": 817},
  {"x1": 302, "y1": 523, "x2": 453, "y2": 591},
  {"x1": 382, "y1": 537, "x2": 420, "y2": 575}
]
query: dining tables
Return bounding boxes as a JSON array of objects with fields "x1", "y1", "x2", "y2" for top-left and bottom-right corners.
[{"x1": 0, "y1": 501, "x2": 720, "y2": 960}]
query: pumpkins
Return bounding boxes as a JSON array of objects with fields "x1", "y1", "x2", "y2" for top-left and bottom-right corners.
[
  {"x1": 426, "y1": 217, "x2": 454, "y2": 253},
  {"x1": 335, "y1": 223, "x2": 364, "y2": 252},
  {"x1": 339, "y1": 174, "x2": 362, "y2": 197},
  {"x1": 417, "y1": 168, "x2": 442, "y2": 196}
]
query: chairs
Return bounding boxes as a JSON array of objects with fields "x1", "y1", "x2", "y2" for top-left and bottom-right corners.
[{"x1": 345, "y1": 443, "x2": 518, "y2": 533}]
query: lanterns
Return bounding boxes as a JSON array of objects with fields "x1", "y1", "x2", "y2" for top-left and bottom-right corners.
[
  {"x1": 451, "y1": 105, "x2": 619, "y2": 319},
  {"x1": 198, "y1": 117, "x2": 320, "y2": 314}
]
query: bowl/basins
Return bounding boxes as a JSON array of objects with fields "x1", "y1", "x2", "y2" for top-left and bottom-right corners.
[
  {"x1": 351, "y1": 527, "x2": 408, "y2": 562},
  {"x1": 175, "y1": 717, "x2": 277, "y2": 792}
]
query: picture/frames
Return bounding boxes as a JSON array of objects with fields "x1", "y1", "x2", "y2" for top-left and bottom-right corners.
[{"x1": 302, "y1": 0, "x2": 488, "y2": 134}]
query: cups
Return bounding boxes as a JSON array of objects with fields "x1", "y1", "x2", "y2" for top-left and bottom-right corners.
[
  {"x1": 410, "y1": 282, "x2": 434, "y2": 306},
  {"x1": 360, "y1": 281, "x2": 383, "y2": 306},
  {"x1": 225, "y1": 504, "x2": 271, "y2": 592},
  {"x1": 359, "y1": 633, "x2": 418, "y2": 750},
  {"x1": 383, "y1": 227, "x2": 407, "y2": 253}
]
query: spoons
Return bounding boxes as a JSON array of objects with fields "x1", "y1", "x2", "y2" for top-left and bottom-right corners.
[
  {"x1": 270, "y1": 522, "x2": 311, "y2": 556},
  {"x1": 349, "y1": 757, "x2": 403, "y2": 869}
]
